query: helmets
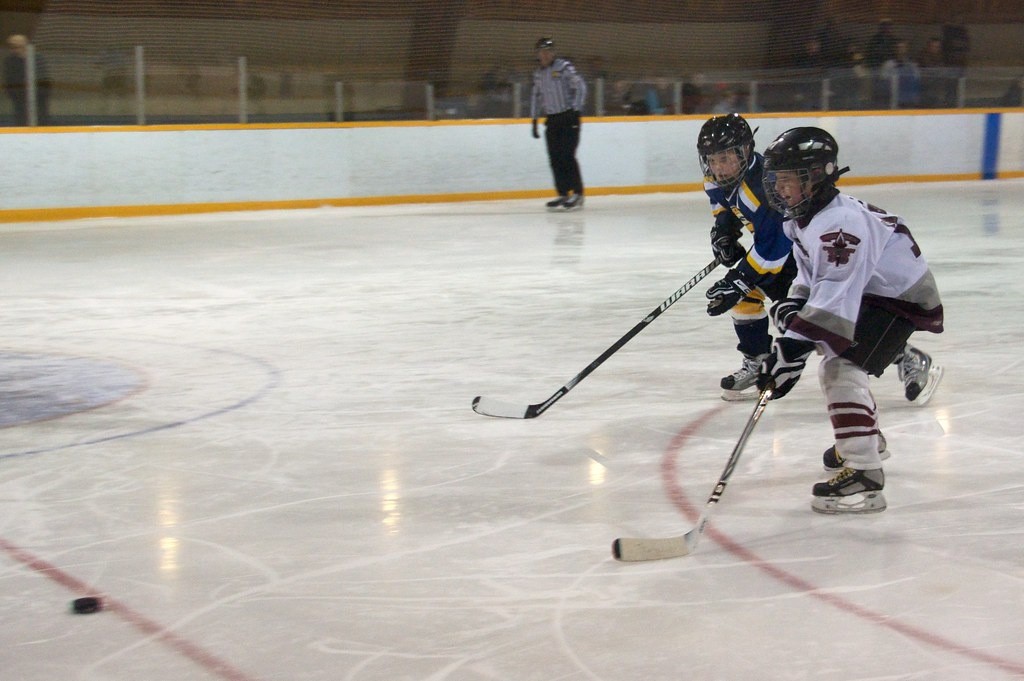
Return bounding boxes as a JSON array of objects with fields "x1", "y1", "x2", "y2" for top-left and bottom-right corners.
[
  {"x1": 763, "y1": 127, "x2": 839, "y2": 216},
  {"x1": 696, "y1": 113, "x2": 754, "y2": 189},
  {"x1": 535, "y1": 38, "x2": 553, "y2": 51}
]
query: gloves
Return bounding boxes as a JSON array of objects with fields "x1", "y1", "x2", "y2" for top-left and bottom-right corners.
[
  {"x1": 769, "y1": 299, "x2": 808, "y2": 334},
  {"x1": 532, "y1": 120, "x2": 539, "y2": 137},
  {"x1": 705, "y1": 269, "x2": 757, "y2": 316},
  {"x1": 710, "y1": 225, "x2": 746, "y2": 267},
  {"x1": 757, "y1": 337, "x2": 815, "y2": 400},
  {"x1": 567, "y1": 111, "x2": 581, "y2": 131}
]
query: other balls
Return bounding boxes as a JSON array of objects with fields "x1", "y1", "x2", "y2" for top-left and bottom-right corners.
[{"x1": 73, "y1": 597, "x2": 98, "y2": 614}]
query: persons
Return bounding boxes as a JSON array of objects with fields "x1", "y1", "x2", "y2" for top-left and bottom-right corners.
[
  {"x1": 697, "y1": 113, "x2": 933, "y2": 402},
  {"x1": 755, "y1": 126, "x2": 944, "y2": 498},
  {"x1": 94, "y1": 17, "x2": 1023, "y2": 120},
  {"x1": 531, "y1": 38, "x2": 586, "y2": 208},
  {"x1": 0, "y1": 35, "x2": 51, "y2": 126}
]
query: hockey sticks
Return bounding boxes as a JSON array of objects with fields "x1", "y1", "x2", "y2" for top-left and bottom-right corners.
[
  {"x1": 609, "y1": 382, "x2": 774, "y2": 562},
  {"x1": 469, "y1": 241, "x2": 741, "y2": 420}
]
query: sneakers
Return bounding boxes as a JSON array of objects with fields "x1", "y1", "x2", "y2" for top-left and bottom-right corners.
[
  {"x1": 890, "y1": 343, "x2": 943, "y2": 406},
  {"x1": 823, "y1": 430, "x2": 891, "y2": 474},
  {"x1": 720, "y1": 335, "x2": 772, "y2": 401},
  {"x1": 810, "y1": 469, "x2": 887, "y2": 514},
  {"x1": 556, "y1": 193, "x2": 582, "y2": 210},
  {"x1": 546, "y1": 196, "x2": 568, "y2": 210}
]
